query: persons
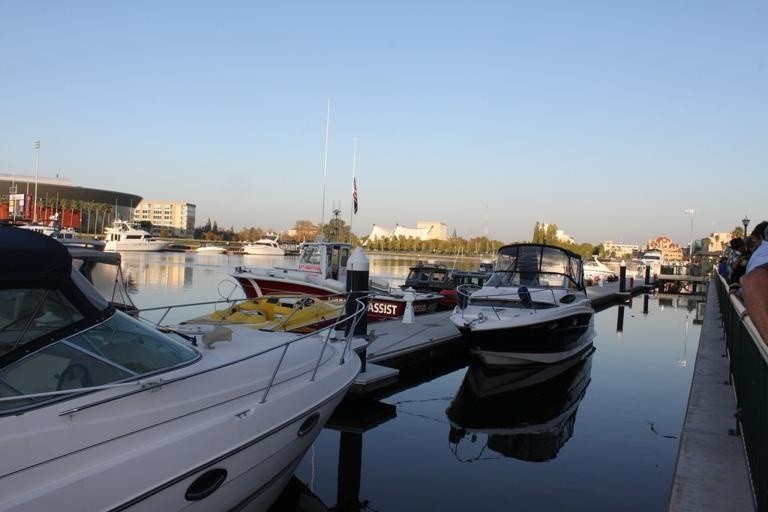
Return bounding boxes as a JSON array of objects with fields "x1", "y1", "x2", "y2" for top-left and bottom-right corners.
[{"x1": 718, "y1": 221, "x2": 768, "y2": 344}]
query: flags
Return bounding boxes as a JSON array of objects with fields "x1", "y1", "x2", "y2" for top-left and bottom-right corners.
[{"x1": 352, "y1": 176, "x2": 361, "y2": 215}]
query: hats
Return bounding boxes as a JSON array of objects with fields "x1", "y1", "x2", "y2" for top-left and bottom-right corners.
[{"x1": 729, "y1": 237, "x2": 745, "y2": 250}]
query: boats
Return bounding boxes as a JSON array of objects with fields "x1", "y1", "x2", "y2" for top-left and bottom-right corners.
[
  {"x1": 449, "y1": 237, "x2": 597, "y2": 370},
  {"x1": 196, "y1": 242, "x2": 228, "y2": 254},
  {"x1": 241, "y1": 232, "x2": 285, "y2": 256},
  {"x1": 445, "y1": 345, "x2": 598, "y2": 463},
  {"x1": 397, "y1": 246, "x2": 459, "y2": 304},
  {"x1": 0, "y1": 218, "x2": 379, "y2": 512},
  {"x1": 103, "y1": 197, "x2": 174, "y2": 251},
  {"x1": 17, "y1": 193, "x2": 74, "y2": 240},
  {"x1": 187, "y1": 290, "x2": 347, "y2": 335},
  {"x1": 539, "y1": 249, "x2": 688, "y2": 286},
  {"x1": 480, "y1": 253, "x2": 498, "y2": 272},
  {"x1": 231, "y1": 97, "x2": 444, "y2": 325}
]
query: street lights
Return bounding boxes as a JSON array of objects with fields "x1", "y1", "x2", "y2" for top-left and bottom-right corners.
[{"x1": 742, "y1": 215, "x2": 751, "y2": 238}]
